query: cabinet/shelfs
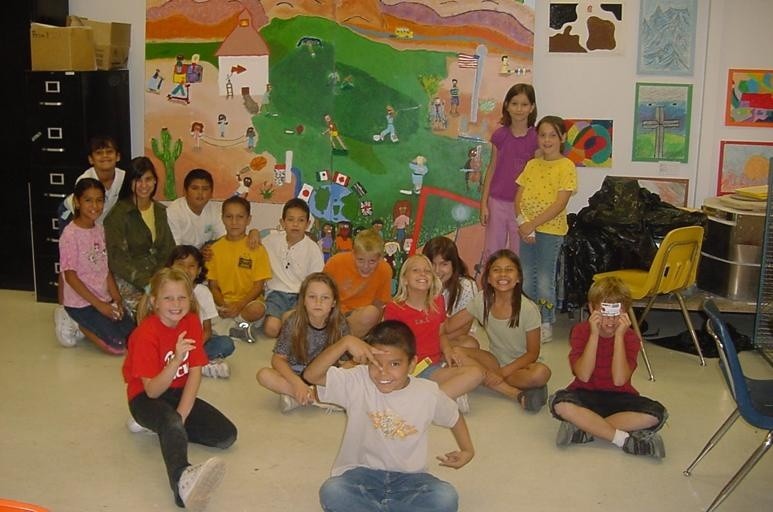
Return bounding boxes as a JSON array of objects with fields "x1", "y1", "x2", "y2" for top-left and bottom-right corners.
[{"x1": 23, "y1": 67, "x2": 130, "y2": 301}]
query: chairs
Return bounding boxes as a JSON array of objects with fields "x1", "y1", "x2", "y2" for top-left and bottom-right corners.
[
  {"x1": 591, "y1": 225, "x2": 707, "y2": 382},
  {"x1": 682, "y1": 296, "x2": 771, "y2": 512}
]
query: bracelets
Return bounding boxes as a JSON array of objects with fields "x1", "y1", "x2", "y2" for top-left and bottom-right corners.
[{"x1": 514, "y1": 214, "x2": 528, "y2": 227}]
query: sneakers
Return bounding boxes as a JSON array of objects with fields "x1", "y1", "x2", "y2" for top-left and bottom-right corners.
[
  {"x1": 540, "y1": 321, "x2": 553, "y2": 344},
  {"x1": 622, "y1": 429, "x2": 665, "y2": 459},
  {"x1": 177, "y1": 456, "x2": 227, "y2": 512},
  {"x1": 53, "y1": 305, "x2": 78, "y2": 347},
  {"x1": 75, "y1": 329, "x2": 84, "y2": 340},
  {"x1": 454, "y1": 393, "x2": 471, "y2": 415},
  {"x1": 279, "y1": 384, "x2": 314, "y2": 413},
  {"x1": 201, "y1": 357, "x2": 231, "y2": 380},
  {"x1": 126, "y1": 415, "x2": 157, "y2": 433},
  {"x1": 556, "y1": 420, "x2": 593, "y2": 446},
  {"x1": 235, "y1": 321, "x2": 257, "y2": 343}
]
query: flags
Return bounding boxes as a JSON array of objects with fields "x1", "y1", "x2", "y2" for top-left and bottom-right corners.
[
  {"x1": 296, "y1": 183, "x2": 313, "y2": 202},
  {"x1": 351, "y1": 182, "x2": 368, "y2": 198},
  {"x1": 332, "y1": 171, "x2": 350, "y2": 187},
  {"x1": 359, "y1": 200, "x2": 372, "y2": 216},
  {"x1": 456, "y1": 53, "x2": 480, "y2": 69},
  {"x1": 314, "y1": 171, "x2": 329, "y2": 181}
]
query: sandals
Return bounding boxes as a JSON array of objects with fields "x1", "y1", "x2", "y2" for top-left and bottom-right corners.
[{"x1": 517, "y1": 383, "x2": 548, "y2": 414}]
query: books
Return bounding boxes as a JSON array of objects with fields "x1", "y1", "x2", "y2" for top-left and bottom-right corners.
[{"x1": 732, "y1": 184, "x2": 772, "y2": 201}]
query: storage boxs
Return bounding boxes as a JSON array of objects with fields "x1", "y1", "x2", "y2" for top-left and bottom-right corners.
[
  {"x1": 67, "y1": 13, "x2": 131, "y2": 71},
  {"x1": 28, "y1": 22, "x2": 98, "y2": 72}
]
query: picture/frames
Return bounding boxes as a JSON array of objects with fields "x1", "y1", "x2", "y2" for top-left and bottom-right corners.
[
  {"x1": 636, "y1": 0, "x2": 698, "y2": 77},
  {"x1": 546, "y1": 2, "x2": 624, "y2": 57},
  {"x1": 723, "y1": 68, "x2": 772, "y2": 128},
  {"x1": 716, "y1": 139, "x2": 773, "y2": 197},
  {"x1": 629, "y1": 82, "x2": 694, "y2": 164},
  {"x1": 615, "y1": 175, "x2": 690, "y2": 209}
]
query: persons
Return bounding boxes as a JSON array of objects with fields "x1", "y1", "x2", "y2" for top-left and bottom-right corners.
[
  {"x1": 441, "y1": 248, "x2": 552, "y2": 413},
  {"x1": 54, "y1": 134, "x2": 393, "y2": 379},
  {"x1": 121, "y1": 266, "x2": 237, "y2": 511},
  {"x1": 549, "y1": 277, "x2": 670, "y2": 459},
  {"x1": 382, "y1": 254, "x2": 485, "y2": 415},
  {"x1": 512, "y1": 115, "x2": 579, "y2": 345},
  {"x1": 301, "y1": 320, "x2": 475, "y2": 511},
  {"x1": 476, "y1": 84, "x2": 543, "y2": 292},
  {"x1": 420, "y1": 236, "x2": 481, "y2": 350},
  {"x1": 256, "y1": 273, "x2": 349, "y2": 414}
]
